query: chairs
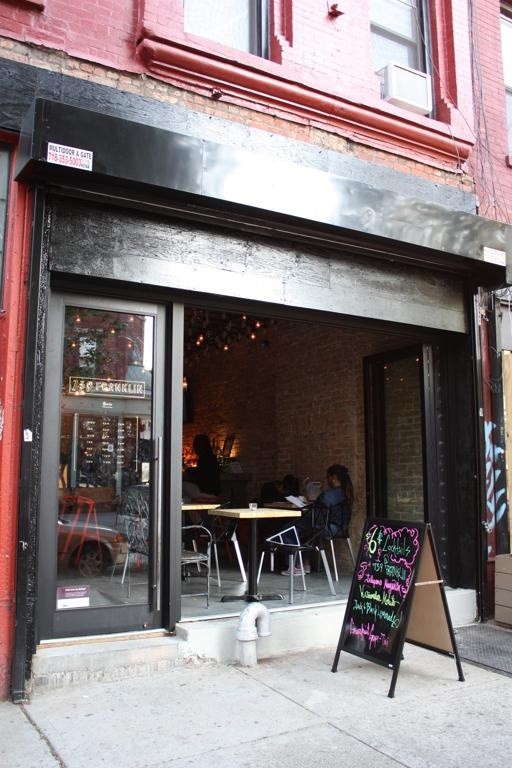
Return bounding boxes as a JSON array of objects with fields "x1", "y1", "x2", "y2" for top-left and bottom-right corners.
[{"x1": 111, "y1": 483, "x2": 357, "y2": 608}]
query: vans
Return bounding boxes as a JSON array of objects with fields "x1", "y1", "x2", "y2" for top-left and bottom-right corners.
[{"x1": 115, "y1": 485, "x2": 150, "y2": 556}]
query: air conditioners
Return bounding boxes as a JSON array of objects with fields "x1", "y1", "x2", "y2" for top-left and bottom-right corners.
[{"x1": 375, "y1": 63, "x2": 432, "y2": 116}]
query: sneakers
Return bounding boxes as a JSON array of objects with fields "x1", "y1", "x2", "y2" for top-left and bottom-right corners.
[{"x1": 281, "y1": 564, "x2": 313, "y2": 577}]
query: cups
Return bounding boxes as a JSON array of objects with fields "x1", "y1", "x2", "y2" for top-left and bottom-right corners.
[{"x1": 248, "y1": 503, "x2": 257, "y2": 512}]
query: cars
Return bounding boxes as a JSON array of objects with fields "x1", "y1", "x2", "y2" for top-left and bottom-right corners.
[{"x1": 57, "y1": 516, "x2": 123, "y2": 577}]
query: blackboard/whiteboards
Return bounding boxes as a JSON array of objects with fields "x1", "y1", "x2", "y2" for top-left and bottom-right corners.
[
  {"x1": 407, "y1": 522, "x2": 459, "y2": 659},
  {"x1": 336, "y1": 519, "x2": 426, "y2": 668}
]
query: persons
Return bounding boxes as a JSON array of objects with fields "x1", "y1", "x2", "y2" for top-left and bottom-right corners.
[
  {"x1": 59, "y1": 431, "x2": 304, "y2": 571},
  {"x1": 270, "y1": 462, "x2": 355, "y2": 577}
]
